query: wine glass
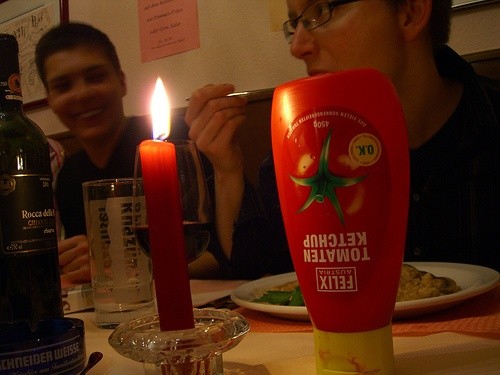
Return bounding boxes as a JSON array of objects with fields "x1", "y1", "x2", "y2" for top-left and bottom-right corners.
[{"x1": 132, "y1": 139, "x2": 210, "y2": 373}]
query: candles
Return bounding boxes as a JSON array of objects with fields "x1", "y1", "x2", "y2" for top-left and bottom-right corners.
[{"x1": 139, "y1": 75, "x2": 195, "y2": 374}]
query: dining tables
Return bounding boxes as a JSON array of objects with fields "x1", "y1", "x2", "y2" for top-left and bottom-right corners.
[{"x1": 64, "y1": 279, "x2": 500, "y2": 375}]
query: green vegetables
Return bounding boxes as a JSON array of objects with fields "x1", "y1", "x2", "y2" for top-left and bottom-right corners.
[{"x1": 252, "y1": 283, "x2": 305, "y2": 306}]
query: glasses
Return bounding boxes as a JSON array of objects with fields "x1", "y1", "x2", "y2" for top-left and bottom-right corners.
[{"x1": 282, "y1": 0, "x2": 361, "y2": 45}]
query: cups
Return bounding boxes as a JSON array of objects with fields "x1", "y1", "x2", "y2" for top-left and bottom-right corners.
[{"x1": 81, "y1": 178, "x2": 154, "y2": 329}]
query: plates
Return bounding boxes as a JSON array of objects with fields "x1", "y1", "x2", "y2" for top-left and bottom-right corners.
[{"x1": 230, "y1": 262, "x2": 500, "y2": 321}]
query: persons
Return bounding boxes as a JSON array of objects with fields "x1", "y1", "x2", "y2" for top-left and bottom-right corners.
[
  {"x1": 35, "y1": 21, "x2": 230, "y2": 283},
  {"x1": 183, "y1": 0, "x2": 500, "y2": 281}
]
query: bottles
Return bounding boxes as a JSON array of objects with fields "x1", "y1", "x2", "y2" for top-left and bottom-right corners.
[
  {"x1": 272, "y1": 69, "x2": 409, "y2": 375},
  {"x1": 0, "y1": 33, "x2": 64, "y2": 346}
]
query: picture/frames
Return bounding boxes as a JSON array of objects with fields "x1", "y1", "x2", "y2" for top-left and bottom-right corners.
[{"x1": 0, "y1": 0, "x2": 69, "y2": 112}]
